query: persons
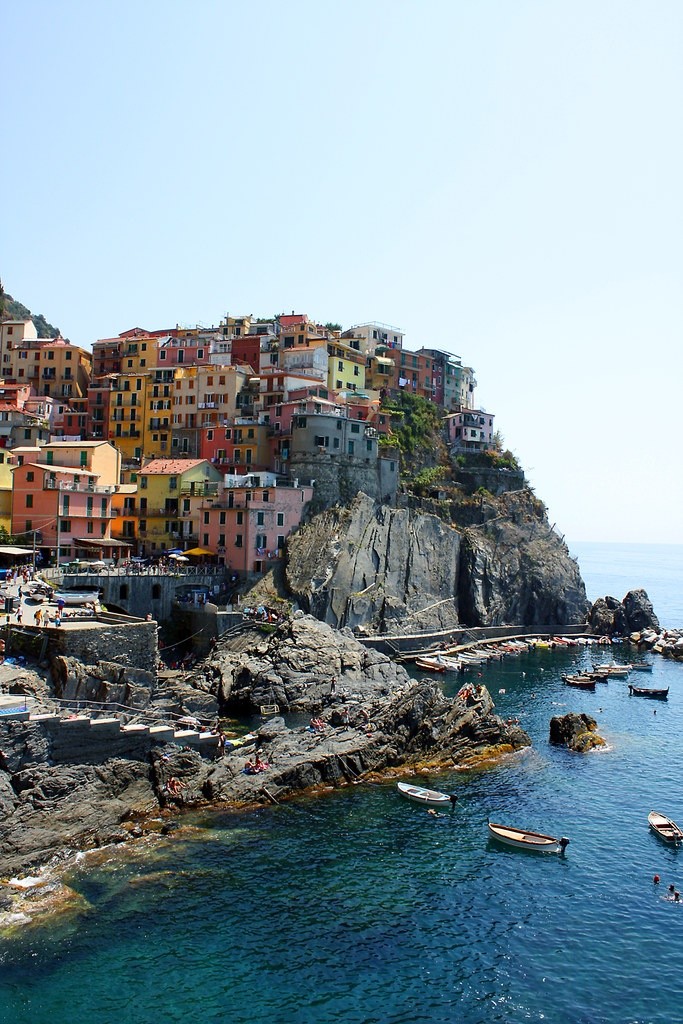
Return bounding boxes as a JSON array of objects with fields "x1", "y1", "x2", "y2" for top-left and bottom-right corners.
[{"x1": 0, "y1": 551, "x2": 556, "y2": 794}]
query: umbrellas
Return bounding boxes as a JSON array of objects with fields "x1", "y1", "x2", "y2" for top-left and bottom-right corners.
[{"x1": 162, "y1": 548, "x2": 214, "y2": 561}]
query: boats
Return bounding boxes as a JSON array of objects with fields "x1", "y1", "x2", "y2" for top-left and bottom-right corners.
[
  {"x1": 397, "y1": 782, "x2": 451, "y2": 807},
  {"x1": 647, "y1": 810, "x2": 683, "y2": 843},
  {"x1": 627, "y1": 684, "x2": 670, "y2": 698},
  {"x1": 562, "y1": 663, "x2": 654, "y2": 685},
  {"x1": 383, "y1": 620, "x2": 625, "y2": 675},
  {"x1": 487, "y1": 822, "x2": 559, "y2": 853}
]
